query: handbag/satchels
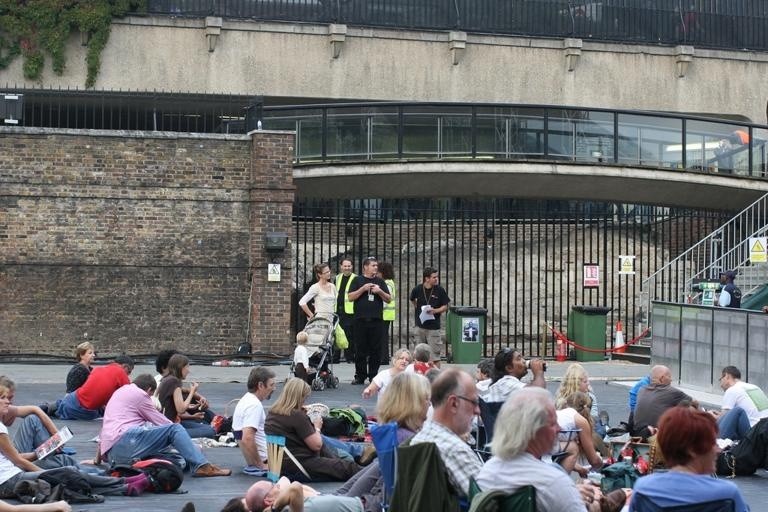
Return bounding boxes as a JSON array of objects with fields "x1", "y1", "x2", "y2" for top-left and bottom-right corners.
[{"x1": 106, "y1": 453, "x2": 188, "y2": 494}]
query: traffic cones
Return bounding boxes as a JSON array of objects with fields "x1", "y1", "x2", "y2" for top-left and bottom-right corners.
[{"x1": 611, "y1": 322, "x2": 627, "y2": 355}]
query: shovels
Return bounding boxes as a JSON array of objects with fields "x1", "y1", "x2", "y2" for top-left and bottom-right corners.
[{"x1": 237, "y1": 275, "x2": 254, "y2": 353}]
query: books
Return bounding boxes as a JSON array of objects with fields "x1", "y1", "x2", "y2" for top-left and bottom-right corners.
[{"x1": 34, "y1": 425, "x2": 76, "y2": 462}]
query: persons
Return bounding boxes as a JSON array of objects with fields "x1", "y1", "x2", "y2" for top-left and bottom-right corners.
[
  {"x1": 409, "y1": 267, "x2": 450, "y2": 370},
  {"x1": 332, "y1": 257, "x2": 360, "y2": 364},
  {"x1": 348, "y1": 256, "x2": 392, "y2": 386},
  {"x1": 78, "y1": 372, "x2": 233, "y2": 477},
  {"x1": 375, "y1": 261, "x2": 396, "y2": 366},
  {"x1": 292, "y1": 330, "x2": 310, "y2": 386},
  {"x1": 714, "y1": 270, "x2": 742, "y2": 310},
  {"x1": 148, "y1": 349, "x2": 221, "y2": 425},
  {"x1": 35, "y1": 354, "x2": 136, "y2": 421},
  {"x1": 298, "y1": 262, "x2": 337, "y2": 321},
  {"x1": 157, "y1": 353, "x2": 234, "y2": 441},
  {"x1": 0, "y1": 498, "x2": 73, "y2": 511},
  {"x1": 65, "y1": 340, "x2": 96, "y2": 393},
  {"x1": 0, "y1": 375, "x2": 116, "y2": 474},
  {"x1": 219, "y1": 342, "x2": 767, "y2": 512},
  {"x1": 0, "y1": 384, "x2": 155, "y2": 496},
  {"x1": 711, "y1": 135, "x2": 736, "y2": 175}
]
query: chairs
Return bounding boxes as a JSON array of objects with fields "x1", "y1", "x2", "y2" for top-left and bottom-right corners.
[
  {"x1": 472, "y1": 394, "x2": 584, "y2": 466},
  {"x1": 366, "y1": 422, "x2": 536, "y2": 511}
]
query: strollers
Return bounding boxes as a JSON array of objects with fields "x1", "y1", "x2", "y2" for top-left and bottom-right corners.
[{"x1": 285, "y1": 310, "x2": 338, "y2": 392}]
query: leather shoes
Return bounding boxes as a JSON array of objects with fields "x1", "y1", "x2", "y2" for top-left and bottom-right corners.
[
  {"x1": 192, "y1": 464, "x2": 232, "y2": 477},
  {"x1": 599, "y1": 409, "x2": 610, "y2": 427},
  {"x1": 357, "y1": 445, "x2": 377, "y2": 466},
  {"x1": 595, "y1": 488, "x2": 627, "y2": 511}
]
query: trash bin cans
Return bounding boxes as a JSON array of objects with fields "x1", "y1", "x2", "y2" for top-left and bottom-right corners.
[
  {"x1": 445, "y1": 304, "x2": 487, "y2": 364},
  {"x1": 566, "y1": 305, "x2": 611, "y2": 361}
]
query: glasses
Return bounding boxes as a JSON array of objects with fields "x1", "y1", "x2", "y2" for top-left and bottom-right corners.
[
  {"x1": 502, "y1": 347, "x2": 510, "y2": 356},
  {"x1": 461, "y1": 395, "x2": 479, "y2": 407},
  {"x1": 367, "y1": 256, "x2": 376, "y2": 261},
  {"x1": 320, "y1": 270, "x2": 331, "y2": 274}
]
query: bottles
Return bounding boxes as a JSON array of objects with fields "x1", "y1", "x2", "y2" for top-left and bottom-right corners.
[{"x1": 525, "y1": 360, "x2": 548, "y2": 372}]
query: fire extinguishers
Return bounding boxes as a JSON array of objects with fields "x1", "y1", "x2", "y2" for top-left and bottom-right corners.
[{"x1": 554, "y1": 333, "x2": 566, "y2": 361}]
